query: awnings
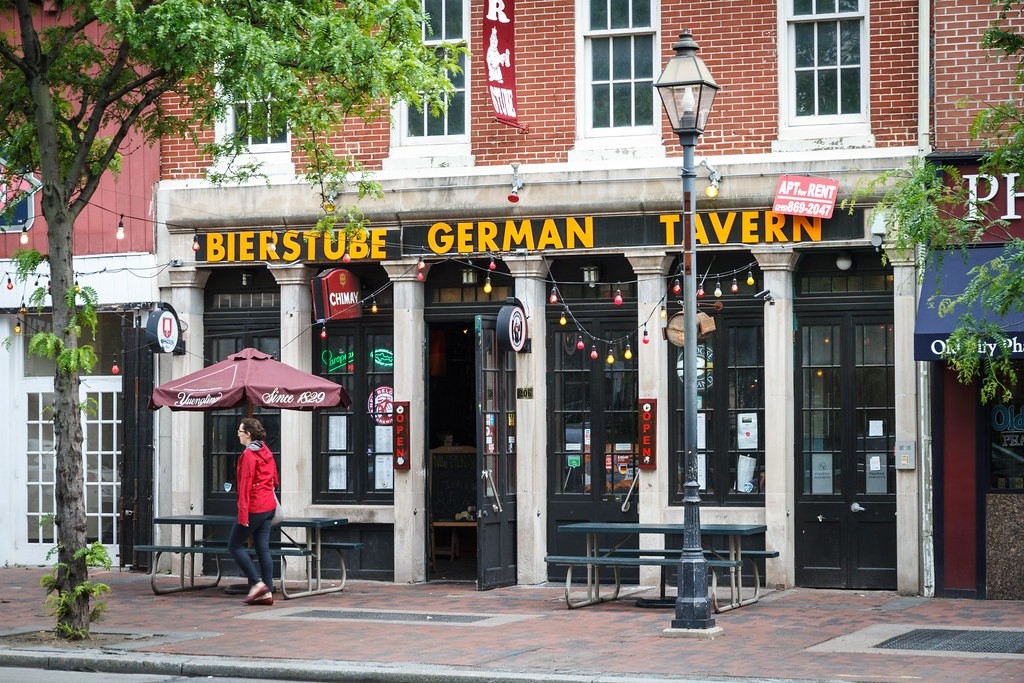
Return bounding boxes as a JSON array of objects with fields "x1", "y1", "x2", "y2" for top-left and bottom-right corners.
[{"x1": 913, "y1": 242, "x2": 1024, "y2": 360}]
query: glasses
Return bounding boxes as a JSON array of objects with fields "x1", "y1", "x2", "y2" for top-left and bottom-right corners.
[{"x1": 238, "y1": 430, "x2": 244, "y2": 434}]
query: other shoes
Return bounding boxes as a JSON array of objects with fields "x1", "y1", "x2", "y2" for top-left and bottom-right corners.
[
  {"x1": 247, "y1": 597, "x2": 273, "y2": 605},
  {"x1": 242, "y1": 585, "x2": 268, "y2": 602}
]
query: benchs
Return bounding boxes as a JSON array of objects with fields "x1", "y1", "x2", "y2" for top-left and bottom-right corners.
[
  {"x1": 542, "y1": 548, "x2": 780, "y2": 568},
  {"x1": 131, "y1": 537, "x2": 363, "y2": 556}
]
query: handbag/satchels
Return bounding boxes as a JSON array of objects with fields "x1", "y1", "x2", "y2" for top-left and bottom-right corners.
[{"x1": 270, "y1": 491, "x2": 284, "y2": 526}]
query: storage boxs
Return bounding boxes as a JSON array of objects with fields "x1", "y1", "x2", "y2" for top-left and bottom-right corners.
[{"x1": 583, "y1": 443, "x2": 638, "y2": 495}]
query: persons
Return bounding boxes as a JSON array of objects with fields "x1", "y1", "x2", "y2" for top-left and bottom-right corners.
[{"x1": 228, "y1": 417, "x2": 279, "y2": 605}]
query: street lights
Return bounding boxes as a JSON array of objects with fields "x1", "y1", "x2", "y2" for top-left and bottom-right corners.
[{"x1": 653, "y1": 28, "x2": 719, "y2": 630}]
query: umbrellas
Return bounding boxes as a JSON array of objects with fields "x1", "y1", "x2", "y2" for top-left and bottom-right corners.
[{"x1": 148, "y1": 346, "x2": 352, "y2": 547}]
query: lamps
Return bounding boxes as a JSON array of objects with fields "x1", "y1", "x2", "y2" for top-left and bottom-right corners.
[
  {"x1": 458, "y1": 267, "x2": 481, "y2": 287},
  {"x1": 696, "y1": 154, "x2": 722, "y2": 200},
  {"x1": 578, "y1": 265, "x2": 601, "y2": 283},
  {"x1": 836, "y1": 252, "x2": 853, "y2": 271},
  {"x1": 507, "y1": 161, "x2": 525, "y2": 203},
  {"x1": 324, "y1": 172, "x2": 340, "y2": 213}
]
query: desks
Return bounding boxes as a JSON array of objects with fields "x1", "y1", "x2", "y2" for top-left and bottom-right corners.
[
  {"x1": 431, "y1": 520, "x2": 479, "y2": 530},
  {"x1": 152, "y1": 512, "x2": 351, "y2": 595},
  {"x1": 554, "y1": 522, "x2": 771, "y2": 613}
]
